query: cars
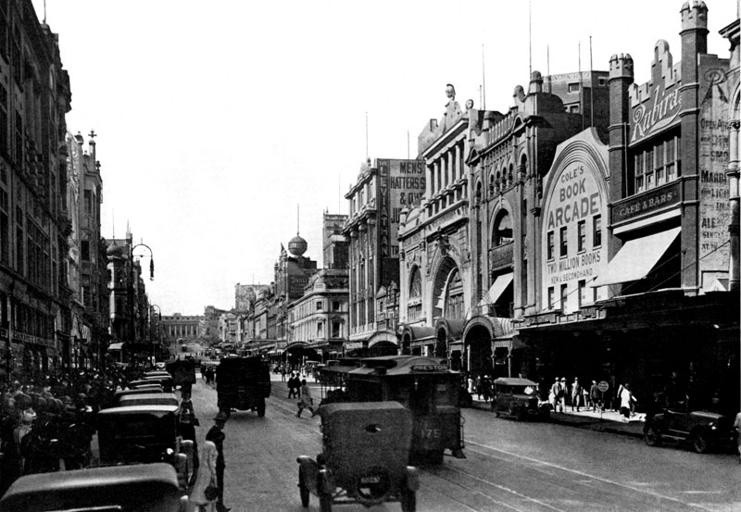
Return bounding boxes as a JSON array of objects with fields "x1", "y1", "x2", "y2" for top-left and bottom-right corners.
[{"x1": 641, "y1": 395, "x2": 738, "y2": 455}]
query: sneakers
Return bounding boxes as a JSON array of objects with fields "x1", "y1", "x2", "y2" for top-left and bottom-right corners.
[
  {"x1": 312, "y1": 415, "x2": 315, "y2": 417},
  {"x1": 295, "y1": 414, "x2": 300, "y2": 417}
]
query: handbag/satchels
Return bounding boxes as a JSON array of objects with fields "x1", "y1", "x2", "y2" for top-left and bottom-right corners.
[{"x1": 204, "y1": 485, "x2": 218, "y2": 500}]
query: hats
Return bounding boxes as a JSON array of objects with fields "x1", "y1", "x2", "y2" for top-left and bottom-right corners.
[{"x1": 213, "y1": 412, "x2": 226, "y2": 421}]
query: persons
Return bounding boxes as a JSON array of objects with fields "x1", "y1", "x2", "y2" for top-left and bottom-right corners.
[
  {"x1": 295, "y1": 379, "x2": 316, "y2": 418},
  {"x1": 294, "y1": 373, "x2": 302, "y2": 398},
  {"x1": 190, "y1": 431, "x2": 227, "y2": 512},
  {"x1": 199, "y1": 365, "x2": 215, "y2": 386},
  {"x1": 205, "y1": 412, "x2": 232, "y2": 512},
  {"x1": 286, "y1": 373, "x2": 298, "y2": 400},
  {"x1": 467, "y1": 370, "x2": 639, "y2": 424}
]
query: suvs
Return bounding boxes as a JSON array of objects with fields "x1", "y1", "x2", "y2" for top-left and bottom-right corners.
[
  {"x1": 491, "y1": 377, "x2": 552, "y2": 421},
  {"x1": 212, "y1": 339, "x2": 466, "y2": 462},
  {"x1": 0, "y1": 340, "x2": 201, "y2": 512},
  {"x1": 295, "y1": 400, "x2": 420, "y2": 511}
]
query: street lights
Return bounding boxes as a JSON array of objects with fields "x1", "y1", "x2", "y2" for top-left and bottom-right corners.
[
  {"x1": 148, "y1": 303, "x2": 161, "y2": 344},
  {"x1": 126, "y1": 243, "x2": 154, "y2": 371}
]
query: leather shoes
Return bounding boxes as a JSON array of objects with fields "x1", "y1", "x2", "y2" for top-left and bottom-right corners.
[{"x1": 218, "y1": 508, "x2": 232, "y2": 511}]
